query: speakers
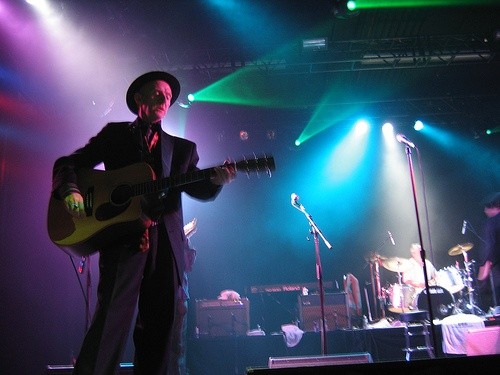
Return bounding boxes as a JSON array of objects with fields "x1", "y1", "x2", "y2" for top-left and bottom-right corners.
[
  {"x1": 268, "y1": 353, "x2": 374, "y2": 369},
  {"x1": 298, "y1": 293, "x2": 349, "y2": 332},
  {"x1": 195, "y1": 298, "x2": 249, "y2": 338}
]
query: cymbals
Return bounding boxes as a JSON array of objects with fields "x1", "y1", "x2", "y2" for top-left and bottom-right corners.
[
  {"x1": 446, "y1": 241, "x2": 475, "y2": 257},
  {"x1": 382, "y1": 256, "x2": 411, "y2": 275},
  {"x1": 363, "y1": 251, "x2": 386, "y2": 266}
]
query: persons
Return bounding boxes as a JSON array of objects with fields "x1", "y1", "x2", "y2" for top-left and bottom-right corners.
[
  {"x1": 403, "y1": 244, "x2": 437, "y2": 291},
  {"x1": 477, "y1": 200, "x2": 500, "y2": 307},
  {"x1": 53, "y1": 71, "x2": 237, "y2": 375}
]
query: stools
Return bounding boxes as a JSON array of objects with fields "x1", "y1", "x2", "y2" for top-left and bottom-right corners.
[{"x1": 399, "y1": 310, "x2": 436, "y2": 362}]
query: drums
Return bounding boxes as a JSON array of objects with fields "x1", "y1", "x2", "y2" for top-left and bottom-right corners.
[
  {"x1": 415, "y1": 285, "x2": 456, "y2": 323},
  {"x1": 435, "y1": 266, "x2": 466, "y2": 295},
  {"x1": 388, "y1": 283, "x2": 414, "y2": 314}
]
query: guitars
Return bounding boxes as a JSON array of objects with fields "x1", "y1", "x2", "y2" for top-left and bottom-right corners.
[{"x1": 46, "y1": 153, "x2": 277, "y2": 259}]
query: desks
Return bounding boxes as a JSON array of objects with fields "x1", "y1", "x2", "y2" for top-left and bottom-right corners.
[{"x1": 441, "y1": 325, "x2": 500, "y2": 356}]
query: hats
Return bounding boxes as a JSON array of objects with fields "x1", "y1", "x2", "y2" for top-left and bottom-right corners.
[{"x1": 126, "y1": 72, "x2": 181, "y2": 116}]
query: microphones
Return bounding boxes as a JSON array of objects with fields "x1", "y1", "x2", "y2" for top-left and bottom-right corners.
[
  {"x1": 388, "y1": 231, "x2": 396, "y2": 246},
  {"x1": 462, "y1": 220, "x2": 467, "y2": 234},
  {"x1": 396, "y1": 134, "x2": 416, "y2": 148},
  {"x1": 289, "y1": 193, "x2": 298, "y2": 200}
]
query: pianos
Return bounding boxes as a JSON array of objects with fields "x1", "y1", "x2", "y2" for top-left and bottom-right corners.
[{"x1": 250, "y1": 282, "x2": 333, "y2": 318}]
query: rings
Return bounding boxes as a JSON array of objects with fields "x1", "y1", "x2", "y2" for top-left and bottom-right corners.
[{"x1": 74, "y1": 203, "x2": 78, "y2": 207}]
